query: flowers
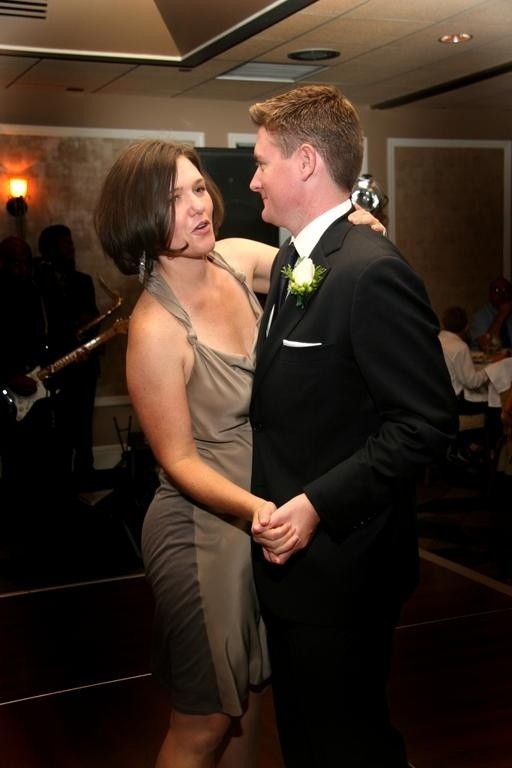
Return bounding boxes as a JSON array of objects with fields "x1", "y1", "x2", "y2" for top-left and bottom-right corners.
[{"x1": 278, "y1": 257, "x2": 326, "y2": 309}]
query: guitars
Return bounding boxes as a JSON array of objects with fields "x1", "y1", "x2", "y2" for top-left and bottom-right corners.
[{"x1": 0, "y1": 317, "x2": 128, "y2": 421}]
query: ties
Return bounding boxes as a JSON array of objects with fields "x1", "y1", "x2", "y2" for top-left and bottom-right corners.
[{"x1": 276, "y1": 242, "x2": 299, "y2": 312}]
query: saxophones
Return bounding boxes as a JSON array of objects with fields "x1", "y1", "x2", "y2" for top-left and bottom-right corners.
[{"x1": 76, "y1": 271, "x2": 125, "y2": 346}]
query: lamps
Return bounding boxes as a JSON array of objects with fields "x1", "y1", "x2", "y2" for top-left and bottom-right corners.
[{"x1": 6, "y1": 179, "x2": 29, "y2": 217}]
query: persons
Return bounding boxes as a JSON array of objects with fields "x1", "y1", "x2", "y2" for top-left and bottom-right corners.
[
  {"x1": 96, "y1": 141, "x2": 391, "y2": 767},
  {"x1": 1, "y1": 236, "x2": 59, "y2": 584},
  {"x1": 252, "y1": 86, "x2": 459, "y2": 768},
  {"x1": 468, "y1": 277, "x2": 512, "y2": 355},
  {"x1": 36, "y1": 224, "x2": 106, "y2": 500},
  {"x1": 436, "y1": 304, "x2": 501, "y2": 463}
]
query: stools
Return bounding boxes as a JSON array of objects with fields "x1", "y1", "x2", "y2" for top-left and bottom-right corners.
[{"x1": 459, "y1": 414, "x2": 492, "y2": 490}]
